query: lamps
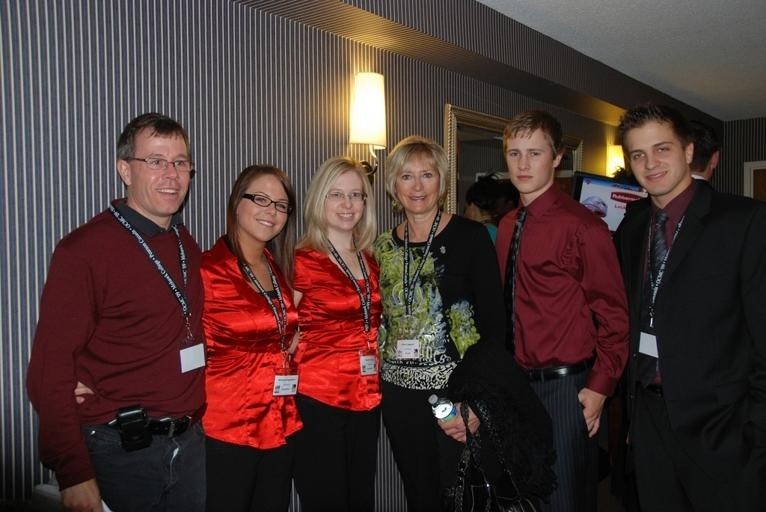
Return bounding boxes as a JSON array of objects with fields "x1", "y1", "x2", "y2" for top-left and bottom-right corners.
[{"x1": 352, "y1": 68, "x2": 389, "y2": 182}]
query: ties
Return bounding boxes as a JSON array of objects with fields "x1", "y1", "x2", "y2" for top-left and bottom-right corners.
[
  {"x1": 503, "y1": 209, "x2": 528, "y2": 351},
  {"x1": 649, "y1": 212, "x2": 668, "y2": 289}
]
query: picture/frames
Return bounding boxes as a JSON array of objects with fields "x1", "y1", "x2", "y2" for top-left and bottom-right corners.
[{"x1": 743, "y1": 161, "x2": 764, "y2": 200}]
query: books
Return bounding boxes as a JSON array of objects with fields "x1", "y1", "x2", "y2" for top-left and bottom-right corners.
[{"x1": 29, "y1": 483, "x2": 112, "y2": 511}]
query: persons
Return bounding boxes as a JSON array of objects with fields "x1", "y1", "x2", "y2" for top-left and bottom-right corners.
[
  {"x1": 368, "y1": 133, "x2": 513, "y2": 511},
  {"x1": 25, "y1": 112, "x2": 209, "y2": 512},
  {"x1": 610, "y1": 102, "x2": 766, "y2": 511},
  {"x1": 494, "y1": 109, "x2": 633, "y2": 511},
  {"x1": 290, "y1": 157, "x2": 381, "y2": 511},
  {"x1": 611, "y1": 119, "x2": 723, "y2": 265},
  {"x1": 461, "y1": 173, "x2": 521, "y2": 248},
  {"x1": 73, "y1": 163, "x2": 301, "y2": 511}
]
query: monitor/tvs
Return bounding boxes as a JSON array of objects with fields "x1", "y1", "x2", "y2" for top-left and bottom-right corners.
[{"x1": 570, "y1": 171, "x2": 649, "y2": 237}]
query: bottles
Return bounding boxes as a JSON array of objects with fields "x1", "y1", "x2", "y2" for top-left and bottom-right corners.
[{"x1": 427, "y1": 393, "x2": 456, "y2": 424}]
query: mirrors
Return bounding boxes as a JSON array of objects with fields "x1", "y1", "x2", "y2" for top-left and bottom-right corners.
[{"x1": 442, "y1": 103, "x2": 519, "y2": 238}]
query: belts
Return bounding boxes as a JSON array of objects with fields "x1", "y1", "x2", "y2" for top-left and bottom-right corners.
[
  {"x1": 522, "y1": 360, "x2": 593, "y2": 389},
  {"x1": 148, "y1": 410, "x2": 205, "y2": 436}
]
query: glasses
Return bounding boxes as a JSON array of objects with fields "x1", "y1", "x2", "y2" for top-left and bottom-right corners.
[
  {"x1": 242, "y1": 193, "x2": 292, "y2": 215},
  {"x1": 126, "y1": 156, "x2": 192, "y2": 170},
  {"x1": 324, "y1": 192, "x2": 367, "y2": 201}
]
